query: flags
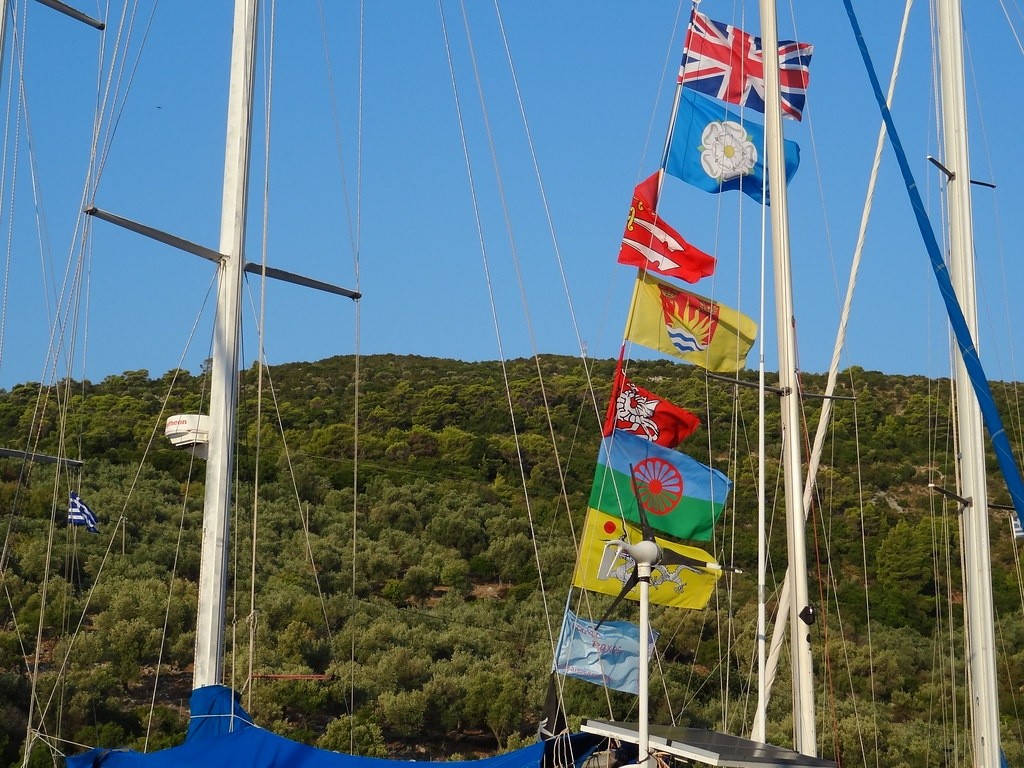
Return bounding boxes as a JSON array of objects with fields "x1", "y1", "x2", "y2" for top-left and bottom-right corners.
[
  {"x1": 68, "y1": 491, "x2": 99, "y2": 534},
  {"x1": 618, "y1": 167, "x2": 717, "y2": 284},
  {"x1": 624, "y1": 268, "x2": 758, "y2": 372},
  {"x1": 677, "y1": 6, "x2": 814, "y2": 122},
  {"x1": 571, "y1": 507, "x2": 723, "y2": 608},
  {"x1": 551, "y1": 609, "x2": 660, "y2": 695},
  {"x1": 591, "y1": 428, "x2": 731, "y2": 542},
  {"x1": 602, "y1": 347, "x2": 702, "y2": 449},
  {"x1": 535, "y1": 674, "x2": 574, "y2": 744},
  {"x1": 660, "y1": 86, "x2": 800, "y2": 206}
]
